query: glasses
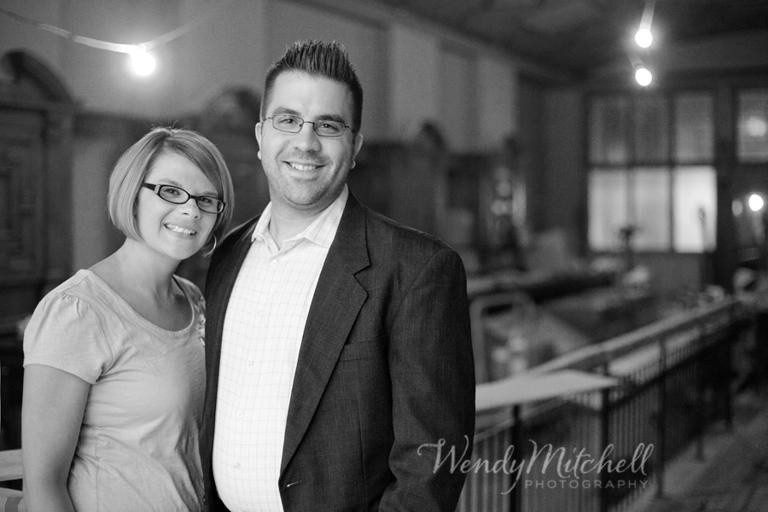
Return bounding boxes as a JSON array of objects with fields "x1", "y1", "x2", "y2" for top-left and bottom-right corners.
[
  {"x1": 262, "y1": 114, "x2": 355, "y2": 137},
  {"x1": 140, "y1": 182, "x2": 225, "y2": 214}
]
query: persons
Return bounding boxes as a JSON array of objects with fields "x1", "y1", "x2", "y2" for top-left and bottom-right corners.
[
  {"x1": 200, "y1": 41, "x2": 478, "y2": 510},
  {"x1": 19, "y1": 128, "x2": 233, "y2": 511}
]
